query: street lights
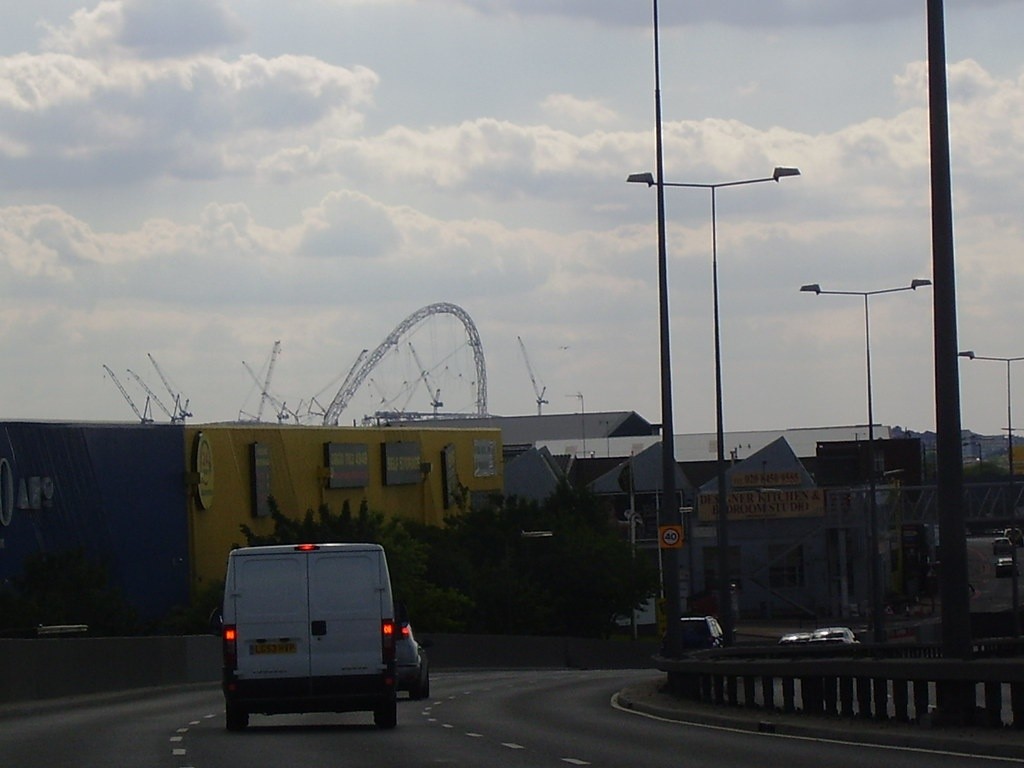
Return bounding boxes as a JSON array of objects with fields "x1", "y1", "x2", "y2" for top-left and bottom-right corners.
[
  {"x1": 627, "y1": 168, "x2": 800, "y2": 646},
  {"x1": 957, "y1": 351, "x2": 1024, "y2": 637},
  {"x1": 502, "y1": 529, "x2": 553, "y2": 669},
  {"x1": 801, "y1": 280, "x2": 931, "y2": 642}
]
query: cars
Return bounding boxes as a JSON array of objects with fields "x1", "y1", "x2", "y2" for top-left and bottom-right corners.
[
  {"x1": 393, "y1": 600, "x2": 433, "y2": 700},
  {"x1": 991, "y1": 527, "x2": 1024, "y2": 578},
  {"x1": 661, "y1": 615, "x2": 725, "y2": 654},
  {"x1": 777, "y1": 626, "x2": 860, "y2": 645}
]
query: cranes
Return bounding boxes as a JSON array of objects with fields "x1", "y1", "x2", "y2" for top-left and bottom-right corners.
[
  {"x1": 239, "y1": 340, "x2": 368, "y2": 426},
  {"x1": 102, "y1": 364, "x2": 155, "y2": 424},
  {"x1": 516, "y1": 336, "x2": 549, "y2": 416},
  {"x1": 370, "y1": 339, "x2": 473, "y2": 420},
  {"x1": 126, "y1": 353, "x2": 192, "y2": 424}
]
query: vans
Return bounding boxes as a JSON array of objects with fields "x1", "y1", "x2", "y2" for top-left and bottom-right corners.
[{"x1": 208, "y1": 543, "x2": 409, "y2": 729}]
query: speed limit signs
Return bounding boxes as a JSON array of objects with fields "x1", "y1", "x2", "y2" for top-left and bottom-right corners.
[{"x1": 658, "y1": 524, "x2": 684, "y2": 548}]
